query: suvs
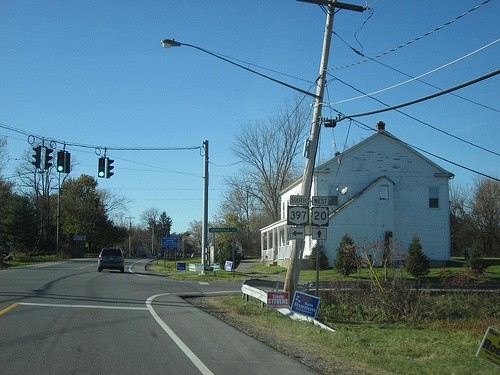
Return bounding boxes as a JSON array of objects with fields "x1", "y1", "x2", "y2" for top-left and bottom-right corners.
[{"x1": 97, "y1": 248, "x2": 124, "y2": 273}]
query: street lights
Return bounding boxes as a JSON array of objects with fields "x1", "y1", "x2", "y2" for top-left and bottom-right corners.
[{"x1": 160, "y1": 39, "x2": 329, "y2": 304}]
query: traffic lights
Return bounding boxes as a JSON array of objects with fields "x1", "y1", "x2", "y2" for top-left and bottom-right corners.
[
  {"x1": 44, "y1": 148, "x2": 53, "y2": 169},
  {"x1": 64, "y1": 153, "x2": 71, "y2": 173},
  {"x1": 56, "y1": 150, "x2": 65, "y2": 172},
  {"x1": 106, "y1": 158, "x2": 114, "y2": 178},
  {"x1": 32, "y1": 146, "x2": 41, "y2": 169},
  {"x1": 98, "y1": 158, "x2": 105, "y2": 177}
]
